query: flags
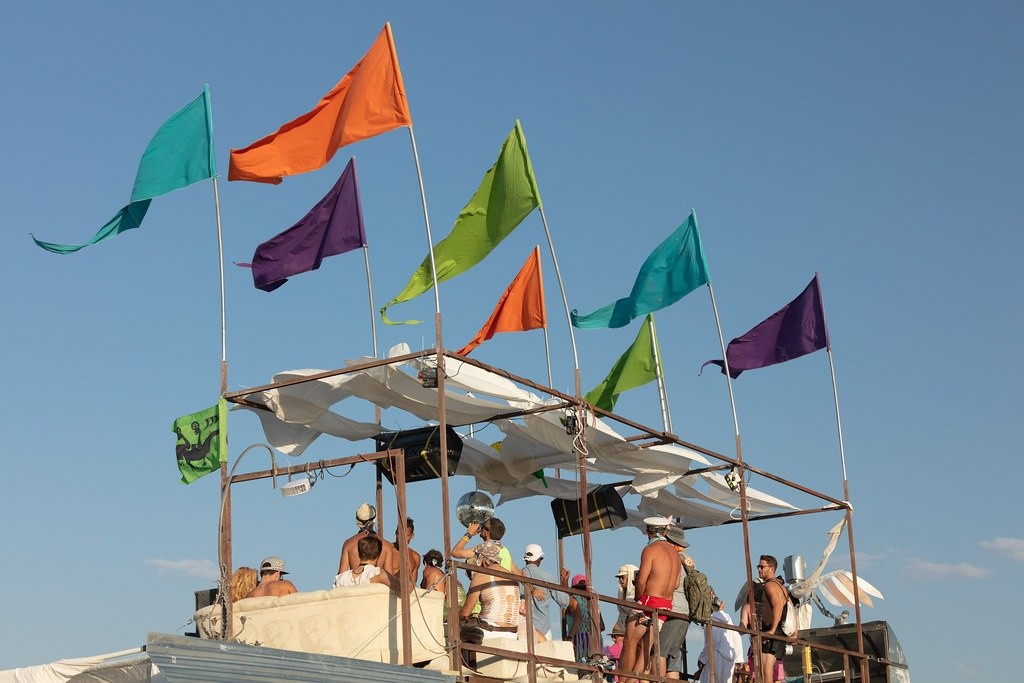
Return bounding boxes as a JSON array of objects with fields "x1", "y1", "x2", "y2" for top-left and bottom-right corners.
[{"x1": 172, "y1": 404, "x2": 221, "y2": 485}]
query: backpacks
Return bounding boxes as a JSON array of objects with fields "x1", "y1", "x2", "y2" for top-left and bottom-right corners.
[{"x1": 765, "y1": 580, "x2": 796, "y2": 637}]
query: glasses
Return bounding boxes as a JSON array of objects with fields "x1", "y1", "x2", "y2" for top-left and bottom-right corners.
[
  {"x1": 757, "y1": 565, "x2": 772, "y2": 569},
  {"x1": 480, "y1": 524, "x2": 490, "y2": 533},
  {"x1": 611, "y1": 635, "x2": 619, "y2": 638},
  {"x1": 540, "y1": 557, "x2": 543, "y2": 560}
]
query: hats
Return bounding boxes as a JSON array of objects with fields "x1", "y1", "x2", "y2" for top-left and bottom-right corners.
[
  {"x1": 356, "y1": 503, "x2": 377, "y2": 528},
  {"x1": 524, "y1": 544, "x2": 545, "y2": 561},
  {"x1": 260, "y1": 557, "x2": 289, "y2": 574},
  {"x1": 666, "y1": 526, "x2": 689, "y2": 548},
  {"x1": 572, "y1": 574, "x2": 586, "y2": 586},
  {"x1": 643, "y1": 517, "x2": 670, "y2": 529},
  {"x1": 606, "y1": 624, "x2": 625, "y2": 635},
  {"x1": 615, "y1": 566, "x2": 629, "y2": 577},
  {"x1": 473, "y1": 541, "x2": 502, "y2": 567}
]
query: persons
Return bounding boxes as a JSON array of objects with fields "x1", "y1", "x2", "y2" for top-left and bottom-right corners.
[
  {"x1": 739, "y1": 555, "x2": 798, "y2": 683},
  {"x1": 520, "y1": 593, "x2": 547, "y2": 643},
  {"x1": 697, "y1": 601, "x2": 743, "y2": 683},
  {"x1": 247, "y1": 557, "x2": 297, "y2": 598},
  {"x1": 618, "y1": 517, "x2": 692, "y2": 683},
  {"x1": 518, "y1": 543, "x2": 570, "y2": 641},
  {"x1": 232, "y1": 567, "x2": 257, "y2": 602},
  {"x1": 566, "y1": 574, "x2": 603, "y2": 662},
  {"x1": 420, "y1": 549, "x2": 444, "y2": 592},
  {"x1": 333, "y1": 536, "x2": 414, "y2": 596},
  {"x1": 602, "y1": 564, "x2": 640, "y2": 682},
  {"x1": 392, "y1": 517, "x2": 420, "y2": 586},
  {"x1": 339, "y1": 503, "x2": 393, "y2": 575},
  {"x1": 453, "y1": 517, "x2": 545, "y2": 614},
  {"x1": 459, "y1": 541, "x2": 520, "y2": 640}
]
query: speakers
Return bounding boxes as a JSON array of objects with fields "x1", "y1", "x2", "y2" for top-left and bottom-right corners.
[
  {"x1": 380, "y1": 426, "x2": 464, "y2": 486},
  {"x1": 551, "y1": 486, "x2": 628, "y2": 537}
]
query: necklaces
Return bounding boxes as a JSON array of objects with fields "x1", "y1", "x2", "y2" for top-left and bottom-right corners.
[{"x1": 359, "y1": 563, "x2": 367, "y2": 566}]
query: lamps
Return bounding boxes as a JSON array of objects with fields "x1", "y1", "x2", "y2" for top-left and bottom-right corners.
[
  {"x1": 559, "y1": 408, "x2": 577, "y2": 435},
  {"x1": 422, "y1": 355, "x2": 446, "y2": 388}
]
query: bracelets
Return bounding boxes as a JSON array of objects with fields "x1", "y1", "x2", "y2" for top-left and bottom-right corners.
[
  {"x1": 531, "y1": 587, "x2": 536, "y2": 593},
  {"x1": 462, "y1": 532, "x2": 471, "y2": 542}
]
